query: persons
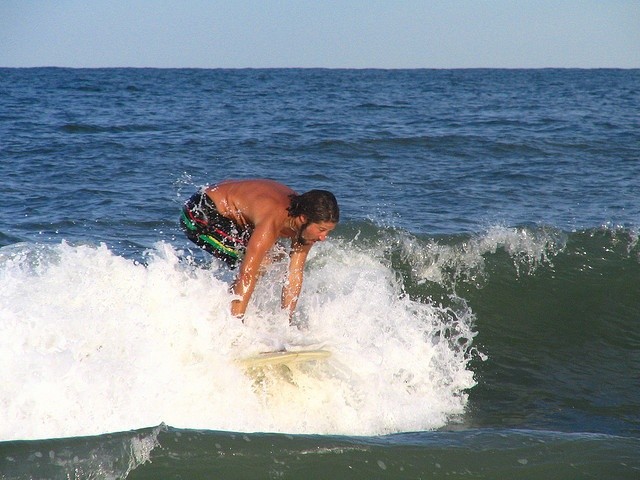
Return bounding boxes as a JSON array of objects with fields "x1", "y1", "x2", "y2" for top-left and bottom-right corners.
[{"x1": 179, "y1": 179, "x2": 339, "y2": 325}]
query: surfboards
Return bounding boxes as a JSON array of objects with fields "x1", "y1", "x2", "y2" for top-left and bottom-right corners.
[{"x1": 241, "y1": 349, "x2": 330, "y2": 368}]
